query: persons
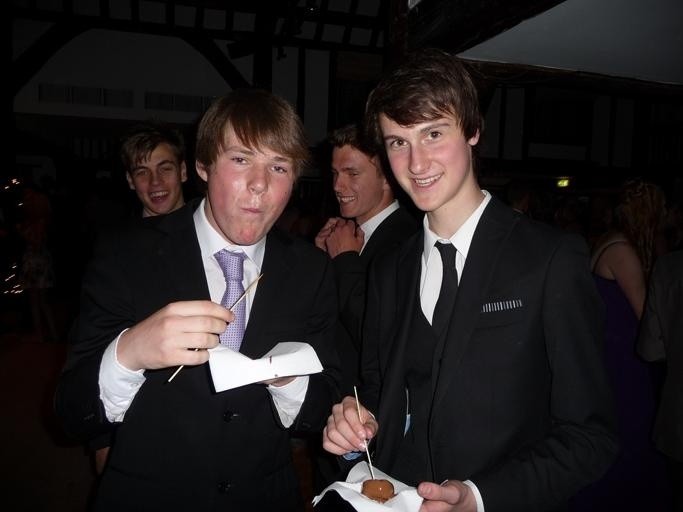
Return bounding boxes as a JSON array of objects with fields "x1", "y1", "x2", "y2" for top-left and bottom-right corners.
[
  {"x1": 322, "y1": 47, "x2": 626, "y2": 510},
  {"x1": 48, "y1": 123, "x2": 189, "y2": 477},
  {"x1": 60, "y1": 85, "x2": 353, "y2": 511},
  {"x1": 310, "y1": 113, "x2": 425, "y2": 511}
]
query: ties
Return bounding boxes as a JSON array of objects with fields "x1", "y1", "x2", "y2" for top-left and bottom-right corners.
[
  {"x1": 431, "y1": 242, "x2": 458, "y2": 334},
  {"x1": 213, "y1": 248, "x2": 248, "y2": 353}
]
